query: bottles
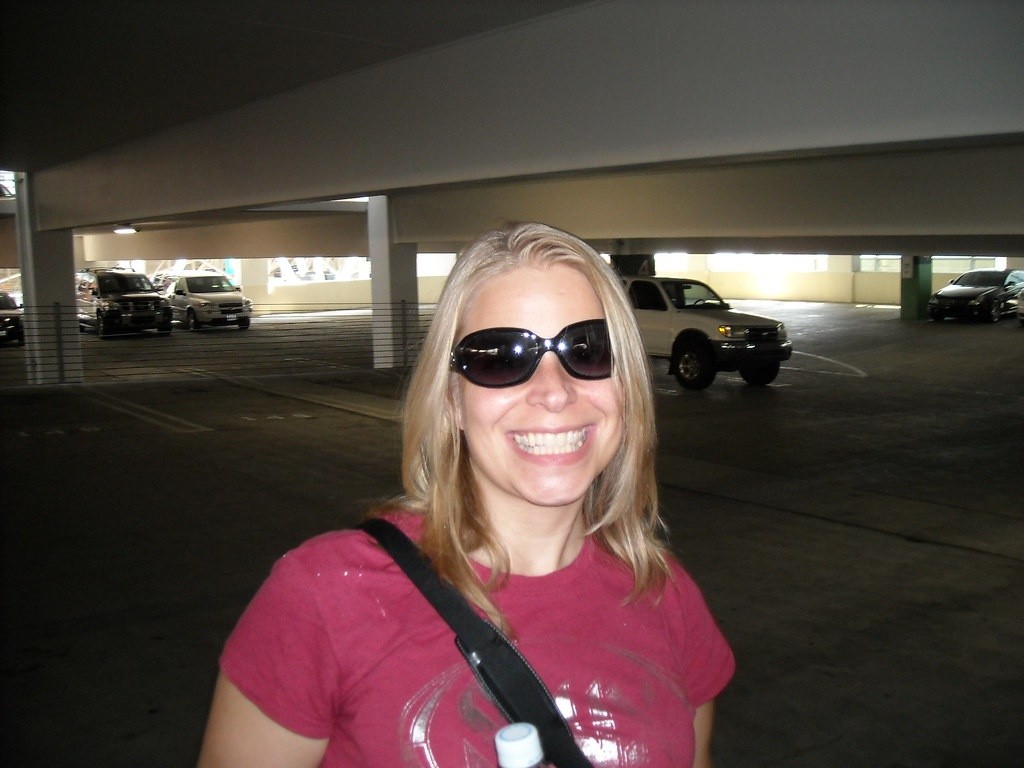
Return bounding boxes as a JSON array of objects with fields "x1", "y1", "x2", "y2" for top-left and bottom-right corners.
[{"x1": 494, "y1": 722, "x2": 548, "y2": 768}]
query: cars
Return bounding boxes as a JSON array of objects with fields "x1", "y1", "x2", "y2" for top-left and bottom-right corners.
[
  {"x1": 0, "y1": 290, "x2": 27, "y2": 347},
  {"x1": 926, "y1": 267, "x2": 1024, "y2": 324}
]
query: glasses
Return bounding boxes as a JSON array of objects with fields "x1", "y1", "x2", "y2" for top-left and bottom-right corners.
[{"x1": 450, "y1": 319, "x2": 613, "y2": 390}]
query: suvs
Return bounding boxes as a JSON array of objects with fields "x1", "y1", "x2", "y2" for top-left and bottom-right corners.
[
  {"x1": 74, "y1": 266, "x2": 175, "y2": 340},
  {"x1": 144, "y1": 268, "x2": 254, "y2": 331},
  {"x1": 614, "y1": 274, "x2": 793, "y2": 391}
]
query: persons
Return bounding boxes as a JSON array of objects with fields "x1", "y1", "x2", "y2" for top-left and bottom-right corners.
[{"x1": 196, "y1": 220, "x2": 735, "y2": 767}]
